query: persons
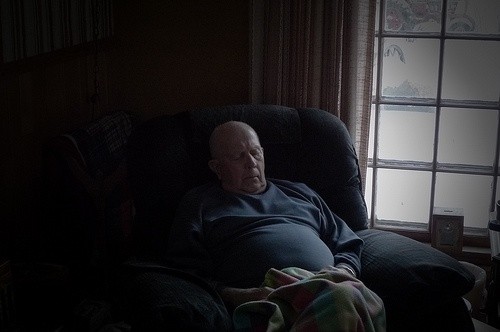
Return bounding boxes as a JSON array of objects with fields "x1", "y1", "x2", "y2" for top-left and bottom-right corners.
[{"x1": 170, "y1": 121, "x2": 386, "y2": 332}]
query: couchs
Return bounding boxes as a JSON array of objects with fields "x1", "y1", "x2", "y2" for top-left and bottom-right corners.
[{"x1": 131, "y1": 104, "x2": 476, "y2": 332}]
